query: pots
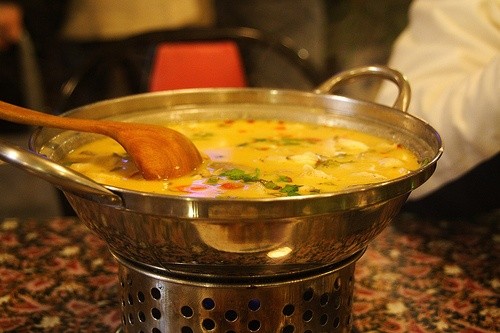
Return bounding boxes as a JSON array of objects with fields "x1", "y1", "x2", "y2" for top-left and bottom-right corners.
[{"x1": 0, "y1": 65, "x2": 445, "y2": 278}]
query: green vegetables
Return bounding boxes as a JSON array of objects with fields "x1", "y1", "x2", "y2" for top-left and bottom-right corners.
[{"x1": 221, "y1": 167, "x2": 304, "y2": 196}]
